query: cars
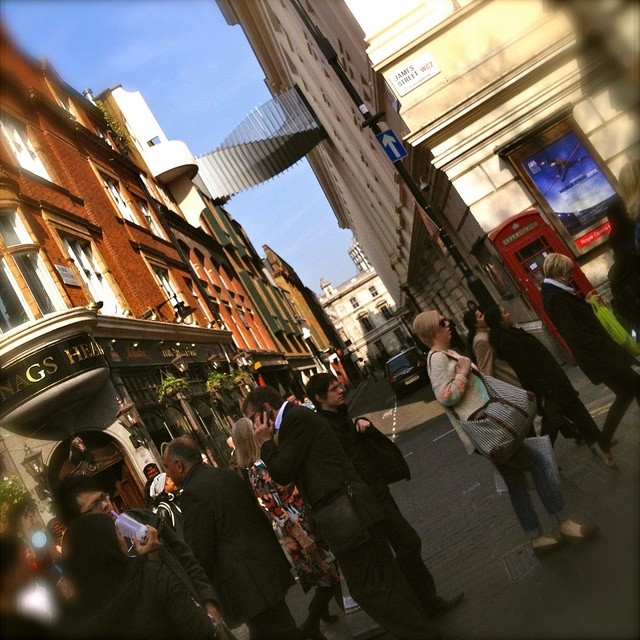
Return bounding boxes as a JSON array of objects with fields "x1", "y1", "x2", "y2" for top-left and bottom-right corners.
[{"x1": 385, "y1": 346, "x2": 430, "y2": 400}]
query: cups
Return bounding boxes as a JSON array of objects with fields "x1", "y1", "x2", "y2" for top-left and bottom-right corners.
[{"x1": 115, "y1": 512, "x2": 149, "y2": 542}]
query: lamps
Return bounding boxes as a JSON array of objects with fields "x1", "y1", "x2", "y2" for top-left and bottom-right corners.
[
  {"x1": 115, "y1": 397, "x2": 149, "y2": 449},
  {"x1": 231, "y1": 352, "x2": 255, "y2": 368},
  {"x1": 157, "y1": 293, "x2": 196, "y2": 325},
  {"x1": 21, "y1": 442, "x2": 51, "y2": 500},
  {"x1": 207, "y1": 350, "x2": 222, "y2": 370},
  {"x1": 170, "y1": 350, "x2": 189, "y2": 373}
]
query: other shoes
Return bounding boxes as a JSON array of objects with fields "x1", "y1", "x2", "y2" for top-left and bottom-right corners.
[
  {"x1": 426, "y1": 592, "x2": 463, "y2": 617},
  {"x1": 589, "y1": 438, "x2": 618, "y2": 470},
  {"x1": 323, "y1": 609, "x2": 339, "y2": 624},
  {"x1": 533, "y1": 539, "x2": 556, "y2": 557},
  {"x1": 559, "y1": 519, "x2": 601, "y2": 539},
  {"x1": 298, "y1": 622, "x2": 327, "y2": 640}
]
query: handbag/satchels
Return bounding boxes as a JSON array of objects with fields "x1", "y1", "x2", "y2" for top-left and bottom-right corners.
[
  {"x1": 591, "y1": 294, "x2": 640, "y2": 356},
  {"x1": 486, "y1": 436, "x2": 561, "y2": 492},
  {"x1": 427, "y1": 349, "x2": 537, "y2": 462},
  {"x1": 308, "y1": 486, "x2": 361, "y2": 545}
]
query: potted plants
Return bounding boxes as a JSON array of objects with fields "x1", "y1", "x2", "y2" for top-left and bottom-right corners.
[
  {"x1": 158, "y1": 369, "x2": 188, "y2": 395},
  {"x1": 206, "y1": 371, "x2": 250, "y2": 393}
]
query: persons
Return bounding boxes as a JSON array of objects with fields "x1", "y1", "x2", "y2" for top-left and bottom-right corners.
[
  {"x1": 0, "y1": 394, "x2": 345, "y2": 640},
  {"x1": 306, "y1": 373, "x2": 463, "y2": 620},
  {"x1": 611, "y1": 158, "x2": 640, "y2": 343},
  {"x1": 242, "y1": 386, "x2": 445, "y2": 640},
  {"x1": 362, "y1": 300, "x2": 619, "y2": 470},
  {"x1": 413, "y1": 309, "x2": 601, "y2": 555},
  {"x1": 542, "y1": 253, "x2": 640, "y2": 470}
]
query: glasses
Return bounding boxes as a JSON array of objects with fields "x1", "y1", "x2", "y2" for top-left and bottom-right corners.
[
  {"x1": 80, "y1": 491, "x2": 106, "y2": 515},
  {"x1": 440, "y1": 318, "x2": 447, "y2": 327}
]
